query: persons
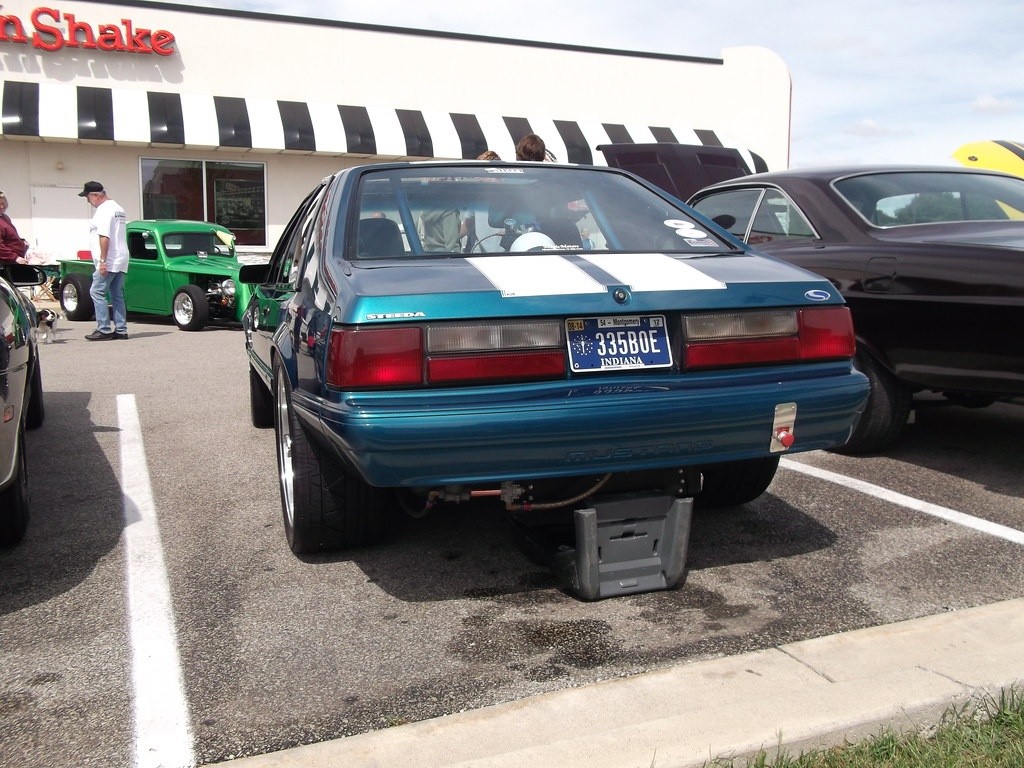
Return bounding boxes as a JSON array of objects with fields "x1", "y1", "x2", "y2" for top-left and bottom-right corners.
[
  {"x1": 461, "y1": 151, "x2": 505, "y2": 253},
  {"x1": 581, "y1": 228, "x2": 595, "y2": 251},
  {"x1": 78, "y1": 181, "x2": 129, "y2": 342},
  {"x1": 416, "y1": 209, "x2": 460, "y2": 253},
  {"x1": 489, "y1": 134, "x2": 567, "y2": 252},
  {"x1": 0, "y1": 190, "x2": 28, "y2": 264}
]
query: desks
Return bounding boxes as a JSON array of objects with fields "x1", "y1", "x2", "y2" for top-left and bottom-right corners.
[{"x1": 28, "y1": 265, "x2": 60, "y2": 302}]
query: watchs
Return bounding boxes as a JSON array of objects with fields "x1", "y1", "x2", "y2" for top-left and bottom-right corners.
[{"x1": 99, "y1": 259, "x2": 105, "y2": 263}]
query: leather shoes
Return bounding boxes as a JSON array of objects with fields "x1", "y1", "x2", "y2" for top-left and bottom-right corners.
[
  {"x1": 111, "y1": 332, "x2": 128, "y2": 339},
  {"x1": 85, "y1": 330, "x2": 112, "y2": 340}
]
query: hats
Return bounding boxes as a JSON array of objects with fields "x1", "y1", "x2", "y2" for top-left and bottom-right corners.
[{"x1": 78, "y1": 181, "x2": 103, "y2": 196}]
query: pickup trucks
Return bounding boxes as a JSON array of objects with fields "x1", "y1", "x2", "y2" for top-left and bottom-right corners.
[{"x1": 56, "y1": 218, "x2": 258, "y2": 331}]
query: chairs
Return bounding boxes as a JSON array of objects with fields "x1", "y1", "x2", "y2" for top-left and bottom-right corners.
[
  {"x1": 357, "y1": 216, "x2": 405, "y2": 257},
  {"x1": 541, "y1": 217, "x2": 582, "y2": 247}
]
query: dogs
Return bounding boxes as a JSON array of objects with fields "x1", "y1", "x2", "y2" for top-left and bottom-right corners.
[{"x1": 36, "y1": 308, "x2": 63, "y2": 344}]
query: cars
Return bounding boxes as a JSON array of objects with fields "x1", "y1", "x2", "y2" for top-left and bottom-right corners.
[
  {"x1": 596, "y1": 144, "x2": 1024, "y2": 455},
  {"x1": 240, "y1": 160, "x2": 872, "y2": 555},
  {"x1": 0, "y1": 264, "x2": 45, "y2": 546}
]
